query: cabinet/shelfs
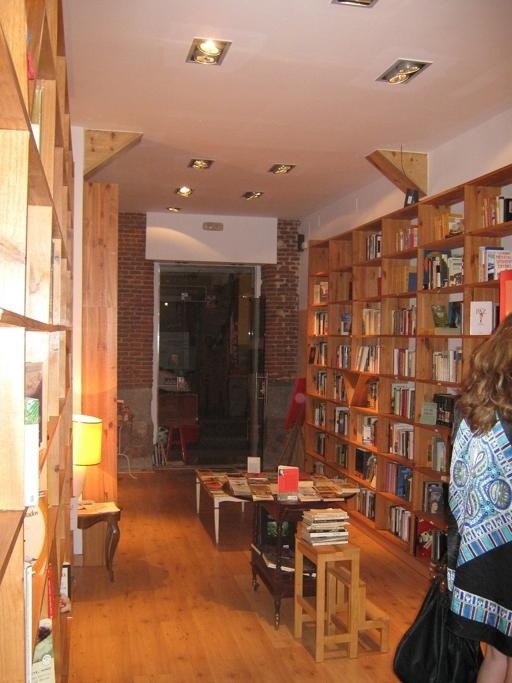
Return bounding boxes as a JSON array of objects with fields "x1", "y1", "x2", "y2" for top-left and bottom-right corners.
[
  {"x1": 305, "y1": 165, "x2": 512, "y2": 575},
  {"x1": 1, "y1": 1, "x2": 75, "y2": 683}
]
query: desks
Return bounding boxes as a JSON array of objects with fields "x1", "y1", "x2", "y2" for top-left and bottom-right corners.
[
  {"x1": 76, "y1": 502, "x2": 120, "y2": 582},
  {"x1": 192, "y1": 468, "x2": 345, "y2": 545},
  {"x1": 221, "y1": 480, "x2": 357, "y2": 631}
]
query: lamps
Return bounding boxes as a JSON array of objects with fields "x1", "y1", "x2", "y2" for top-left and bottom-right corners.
[{"x1": 72, "y1": 414, "x2": 103, "y2": 497}]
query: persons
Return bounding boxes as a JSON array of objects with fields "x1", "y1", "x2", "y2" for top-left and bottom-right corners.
[{"x1": 440, "y1": 307, "x2": 511, "y2": 682}]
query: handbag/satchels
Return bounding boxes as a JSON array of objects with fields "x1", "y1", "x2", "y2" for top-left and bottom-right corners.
[{"x1": 392, "y1": 573, "x2": 484, "y2": 683}]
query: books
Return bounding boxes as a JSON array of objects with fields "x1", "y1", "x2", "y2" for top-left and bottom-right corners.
[
  {"x1": 31, "y1": 618, "x2": 56, "y2": 683},
  {"x1": 61, "y1": 560, "x2": 72, "y2": 614},
  {"x1": 24, "y1": 393, "x2": 40, "y2": 508},
  {"x1": 196, "y1": 452, "x2": 309, "y2": 504},
  {"x1": 251, "y1": 542, "x2": 296, "y2": 557},
  {"x1": 261, "y1": 551, "x2": 317, "y2": 578},
  {"x1": 294, "y1": 507, "x2": 350, "y2": 548}
]
